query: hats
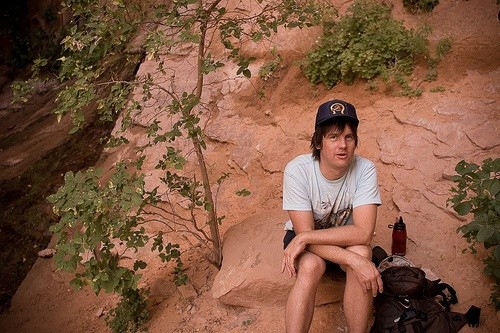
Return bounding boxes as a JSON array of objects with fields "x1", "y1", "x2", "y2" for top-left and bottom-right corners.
[{"x1": 314, "y1": 99, "x2": 359, "y2": 127}]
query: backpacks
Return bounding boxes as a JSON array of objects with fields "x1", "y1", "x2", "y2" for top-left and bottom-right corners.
[{"x1": 370, "y1": 255, "x2": 467, "y2": 333}]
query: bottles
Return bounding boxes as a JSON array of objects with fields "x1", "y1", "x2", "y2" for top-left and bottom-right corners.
[{"x1": 392, "y1": 217, "x2": 406, "y2": 255}]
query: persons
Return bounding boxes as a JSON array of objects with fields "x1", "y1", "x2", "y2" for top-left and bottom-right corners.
[{"x1": 282, "y1": 99, "x2": 383, "y2": 333}]
